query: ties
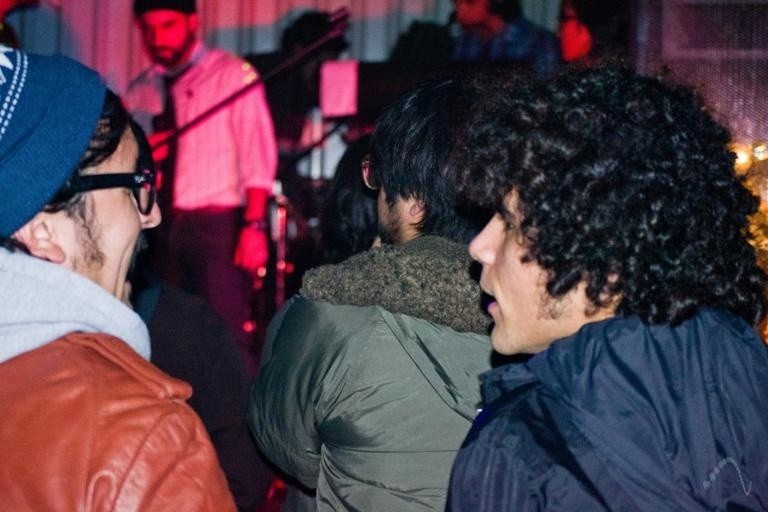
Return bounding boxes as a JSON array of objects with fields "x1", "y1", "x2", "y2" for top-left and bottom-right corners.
[{"x1": 160, "y1": 63, "x2": 190, "y2": 217}]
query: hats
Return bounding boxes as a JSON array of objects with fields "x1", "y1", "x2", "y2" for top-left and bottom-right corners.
[{"x1": 0, "y1": 45, "x2": 105, "y2": 242}]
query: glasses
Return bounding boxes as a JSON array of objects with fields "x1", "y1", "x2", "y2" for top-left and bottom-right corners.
[
  {"x1": 362, "y1": 153, "x2": 386, "y2": 190},
  {"x1": 68, "y1": 149, "x2": 155, "y2": 217}
]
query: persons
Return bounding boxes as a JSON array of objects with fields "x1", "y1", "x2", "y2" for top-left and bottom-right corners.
[
  {"x1": 246, "y1": 9, "x2": 348, "y2": 219},
  {"x1": 451, "y1": 66, "x2": 768, "y2": 512},
  {"x1": 445, "y1": 2, "x2": 560, "y2": 76},
  {"x1": 320, "y1": 135, "x2": 382, "y2": 262},
  {"x1": 1, "y1": 44, "x2": 238, "y2": 511},
  {"x1": 249, "y1": 60, "x2": 547, "y2": 512},
  {"x1": 132, "y1": 275, "x2": 275, "y2": 511},
  {"x1": 125, "y1": 0, "x2": 278, "y2": 362}
]
